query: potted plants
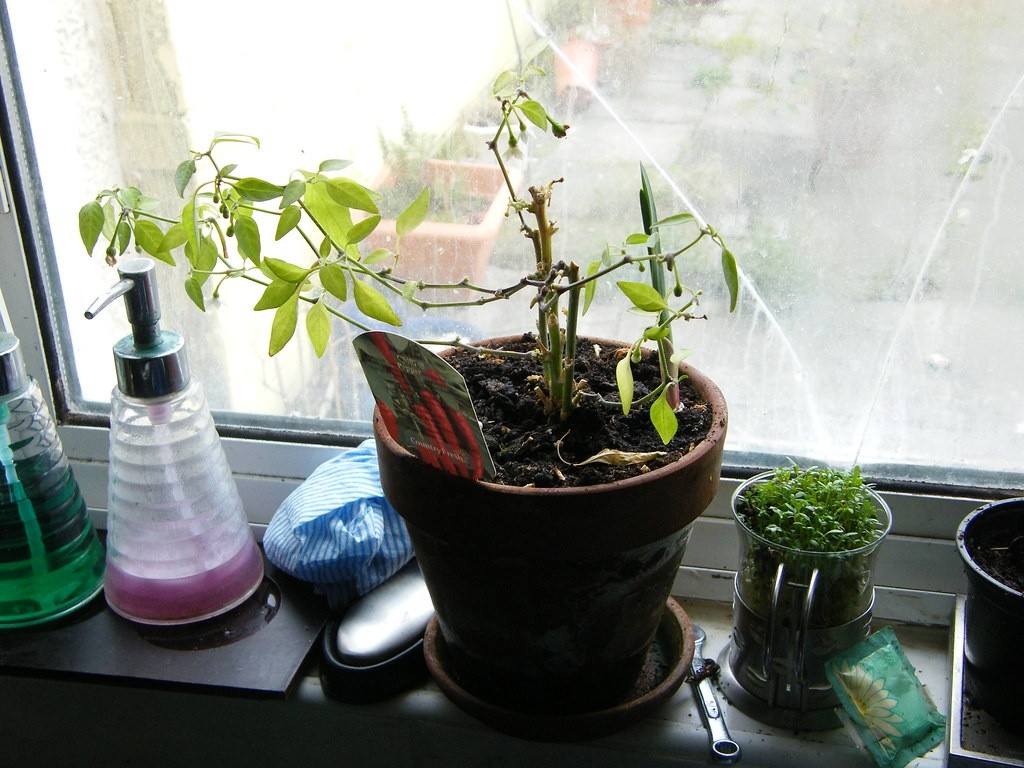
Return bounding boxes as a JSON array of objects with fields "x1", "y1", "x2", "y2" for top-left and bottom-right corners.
[
  {"x1": 77, "y1": 63, "x2": 736, "y2": 705},
  {"x1": 730, "y1": 463, "x2": 893, "y2": 727}
]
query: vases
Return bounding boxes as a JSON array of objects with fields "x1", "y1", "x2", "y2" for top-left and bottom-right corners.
[{"x1": 954, "y1": 495, "x2": 1024, "y2": 709}]
